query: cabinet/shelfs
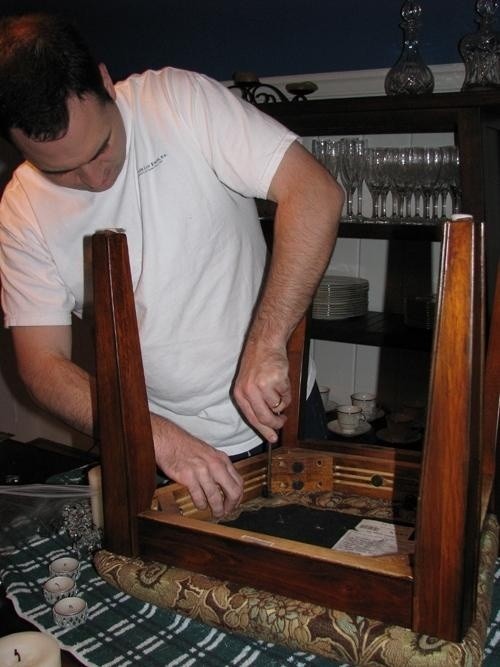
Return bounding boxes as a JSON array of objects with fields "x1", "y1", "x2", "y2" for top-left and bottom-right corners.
[{"x1": 252, "y1": 85, "x2": 500, "y2": 452}]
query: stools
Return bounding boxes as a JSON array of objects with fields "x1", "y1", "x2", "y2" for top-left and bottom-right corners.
[{"x1": 86, "y1": 215, "x2": 498, "y2": 666}]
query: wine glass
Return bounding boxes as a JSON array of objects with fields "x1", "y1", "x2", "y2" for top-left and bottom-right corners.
[{"x1": 310, "y1": 134, "x2": 462, "y2": 229}]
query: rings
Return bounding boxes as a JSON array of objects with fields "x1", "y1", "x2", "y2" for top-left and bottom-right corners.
[{"x1": 273, "y1": 398, "x2": 282, "y2": 408}]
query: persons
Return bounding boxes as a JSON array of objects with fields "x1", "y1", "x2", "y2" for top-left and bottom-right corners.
[{"x1": 1, "y1": 32, "x2": 347, "y2": 519}]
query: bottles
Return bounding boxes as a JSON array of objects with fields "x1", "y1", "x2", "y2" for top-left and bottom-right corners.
[
  {"x1": 380, "y1": 4, "x2": 436, "y2": 96},
  {"x1": 460, "y1": 0, "x2": 500, "y2": 91}
]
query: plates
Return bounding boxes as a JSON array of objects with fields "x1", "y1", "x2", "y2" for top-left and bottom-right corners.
[
  {"x1": 321, "y1": 400, "x2": 341, "y2": 414},
  {"x1": 367, "y1": 411, "x2": 388, "y2": 422},
  {"x1": 312, "y1": 274, "x2": 369, "y2": 320},
  {"x1": 375, "y1": 428, "x2": 422, "y2": 446},
  {"x1": 402, "y1": 296, "x2": 437, "y2": 330},
  {"x1": 329, "y1": 419, "x2": 373, "y2": 435}
]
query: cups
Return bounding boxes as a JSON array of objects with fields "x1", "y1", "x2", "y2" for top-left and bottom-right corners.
[
  {"x1": 349, "y1": 392, "x2": 385, "y2": 415},
  {"x1": 338, "y1": 403, "x2": 367, "y2": 432},
  {"x1": 318, "y1": 385, "x2": 330, "y2": 405},
  {"x1": 386, "y1": 414, "x2": 415, "y2": 439}
]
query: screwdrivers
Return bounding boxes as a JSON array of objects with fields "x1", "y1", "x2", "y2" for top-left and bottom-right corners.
[{"x1": 267, "y1": 412, "x2": 280, "y2": 497}]
